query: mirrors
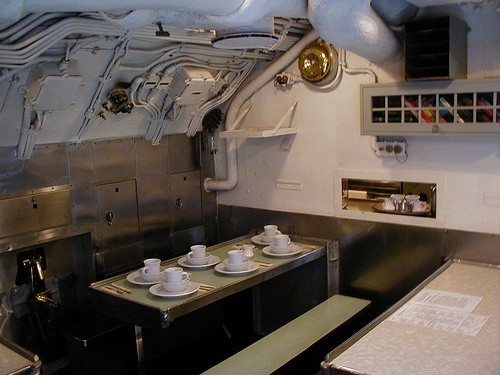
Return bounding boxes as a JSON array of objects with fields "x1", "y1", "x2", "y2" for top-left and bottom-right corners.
[{"x1": 339, "y1": 177, "x2": 437, "y2": 219}]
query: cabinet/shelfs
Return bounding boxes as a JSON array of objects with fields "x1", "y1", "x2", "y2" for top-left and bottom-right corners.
[{"x1": 404, "y1": 14, "x2": 470, "y2": 81}]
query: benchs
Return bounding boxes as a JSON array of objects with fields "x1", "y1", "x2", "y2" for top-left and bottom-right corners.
[{"x1": 200, "y1": 294, "x2": 371, "y2": 375}]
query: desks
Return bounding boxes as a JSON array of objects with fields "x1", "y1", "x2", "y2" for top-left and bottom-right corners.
[
  {"x1": 88, "y1": 231, "x2": 339, "y2": 375},
  {"x1": 320, "y1": 258, "x2": 500, "y2": 375}
]
